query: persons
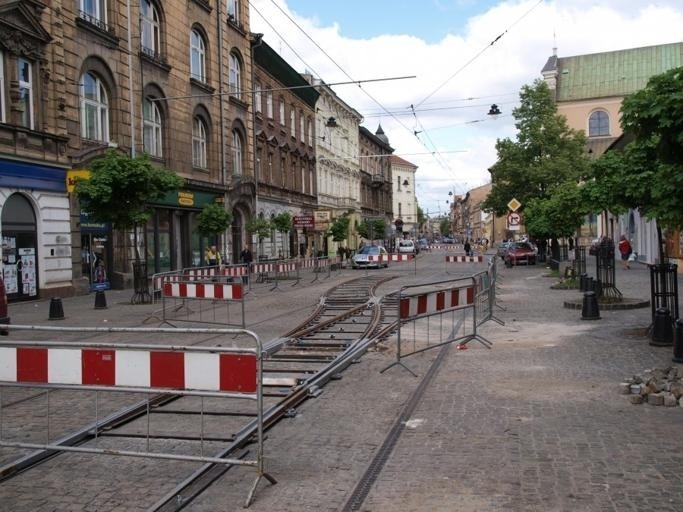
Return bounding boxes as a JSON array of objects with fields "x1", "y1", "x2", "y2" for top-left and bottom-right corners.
[
  {"x1": 464, "y1": 240, "x2": 471, "y2": 255},
  {"x1": 205, "y1": 245, "x2": 222, "y2": 282},
  {"x1": 475, "y1": 238, "x2": 481, "y2": 245},
  {"x1": 238, "y1": 245, "x2": 252, "y2": 285},
  {"x1": 618, "y1": 234, "x2": 632, "y2": 270}
]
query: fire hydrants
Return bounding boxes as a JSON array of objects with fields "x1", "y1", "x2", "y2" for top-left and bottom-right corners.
[{"x1": 0, "y1": 277, "x2": 14, "y2": 333}]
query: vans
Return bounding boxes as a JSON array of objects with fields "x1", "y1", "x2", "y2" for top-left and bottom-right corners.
[{"x1": 397, "y1": 239, "x2": 418, "y2": 255}]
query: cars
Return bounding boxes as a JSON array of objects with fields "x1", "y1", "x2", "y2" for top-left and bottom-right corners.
[
  {"x1": 415, "y1": 233, "x2": 456, "y2": 250},
  {"x1": 350, "y1": 243, "x2": 392, "y2": 269},
  {"x1": 589, "y1": 238, "x2": 600, "y2": 255},
  {"x1": 501, "y1": 236, "x2": 540, "y2": 268}
]
